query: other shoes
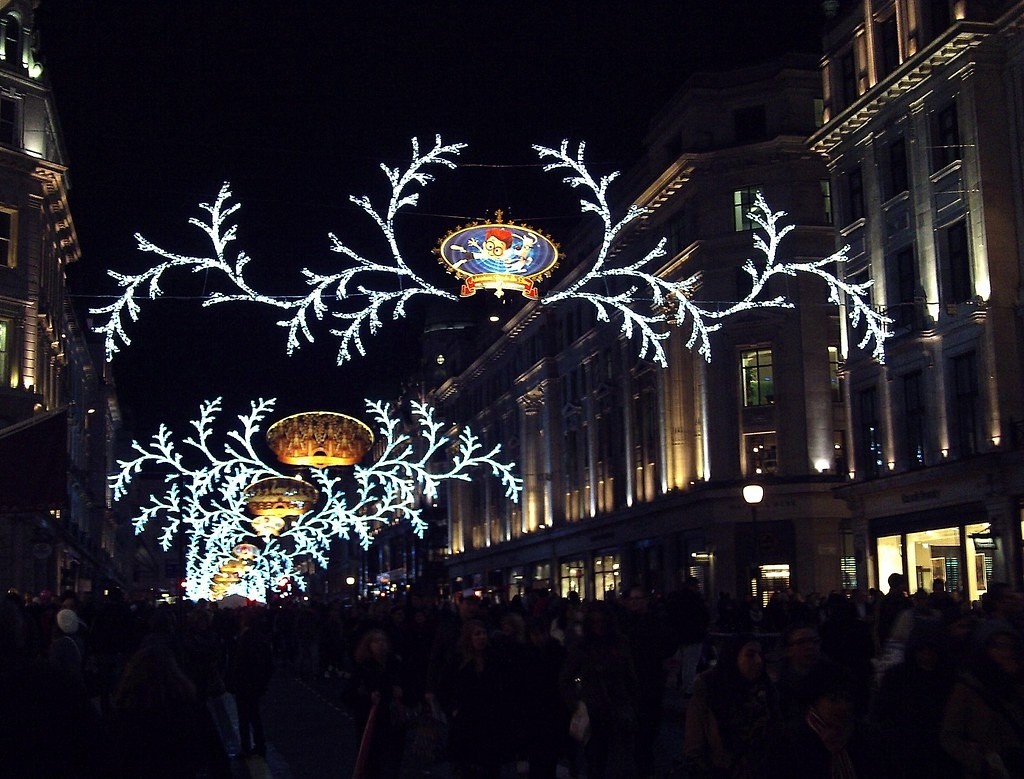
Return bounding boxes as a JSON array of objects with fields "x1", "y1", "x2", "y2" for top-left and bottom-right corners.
[
  {"x1": 235, "y1": 750, "x2": 251, "y2": 760},
  {"x1": 251, "y1": 745, "x2": 267, "y2": 759}
]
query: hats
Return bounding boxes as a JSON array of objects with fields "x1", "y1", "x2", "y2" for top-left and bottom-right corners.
[
  {"x1": 56, "y1": 608, "x2": 79, "y2": 634},
  {"x1": 976, "y1": 618, "x2": 1023, "y2": 654}
]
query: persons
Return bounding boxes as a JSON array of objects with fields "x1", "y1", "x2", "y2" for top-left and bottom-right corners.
[
  {"x1": 985, "y1": 631, "x2": 1024, "y2": 704},
  {"x1": 559, "y1": 602, "x2": 635, "y2": 779},
  {"x1": 342, "y1": 629, "x2": 419, "y2": 779},
  {"x1": 940, "y1": 675, "x2": 1024, "y2": 779},
  {"x1": 684, "y1": 633, "x2": 776, "y2": 779},
  {"x1": 763, "y1": 665, "x2": 872, "y2": 779},
  {"x1": 0, "y1": 574, "x2": 1024, "y2": 779},
  {"x1": 777, "y1": 622, "x2": 833, "y2": 707},
  {"x1": 439, "y1": 620, "x2": 507, "y2": 778}
]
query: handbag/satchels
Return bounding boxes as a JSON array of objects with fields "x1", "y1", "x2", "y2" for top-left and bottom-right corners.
[{"x1": 570, "y1": 699, "x2": 593, "y2": 748}]
